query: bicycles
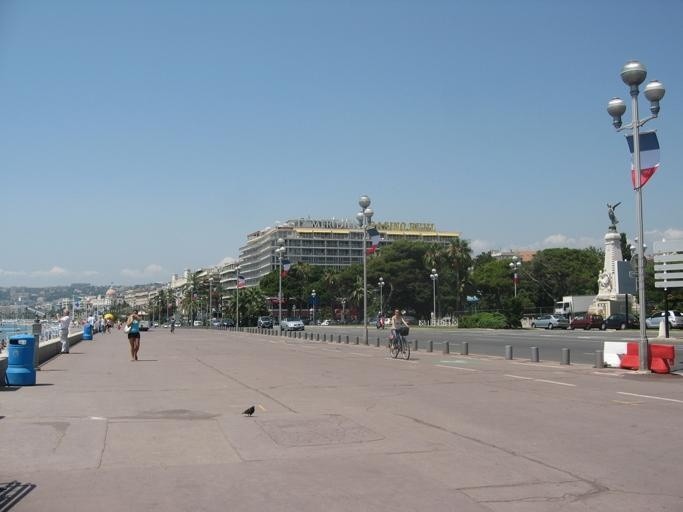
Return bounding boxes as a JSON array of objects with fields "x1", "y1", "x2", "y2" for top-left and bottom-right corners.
[{"x1": 388, "y1": 326, "x2": 412, "y2": 360}]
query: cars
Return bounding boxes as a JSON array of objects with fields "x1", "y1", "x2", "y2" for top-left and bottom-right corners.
[
  {"x1": 530, "y1": 309, "x2": 683, "y2": 330},
  {"x1": 194, "y1": 321, "x2": 204, "y2": 326},
  {"x1": 213, "y1": 318, "x2": 234, "y2": 326},
  {"x1": 279, "y1": 318, "x2": 305, "y2": 330},
  {"x1": 257, "y1": 316, "x2": 273, "y2": 328}
]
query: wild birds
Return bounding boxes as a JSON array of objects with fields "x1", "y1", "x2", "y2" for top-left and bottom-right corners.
[{"x1": 241, "y1": 406, "x2": 255, "y2": 417}]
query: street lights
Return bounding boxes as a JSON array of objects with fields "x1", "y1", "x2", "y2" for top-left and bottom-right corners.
[
  {"x1": 510, "y1": 255, "x2": 522, "y2": 296},
  {"x1": 429, "y1": 269, "x2": 438, "y2": 322},
  {"x1": 276, "y1": 238, "x2": 287, "y2": 328},
  {"x1": 234, "y1": 260, "x2": 241, "y2": 328},
  {"x1": 606, "y1": 60, "x2": 666, "y2": 374},
  {"x1": 378, "y1": 276, "x2": 384, "y2": 312},
  {"x1": 292, "y1": 305, "x2": 297, "y2": 317},
  {"x1": 356, "y1": 194, "x2": 374, "y2": 345},
  {"x1": 311, "y1": 290, "x2": 315, "y2": 318},
  {"x1": 341, "y1": 299, "x2": 346, "y2": 320},
  {"x1": 631, "y1": 237, "x2": 648, "y2": 294}
]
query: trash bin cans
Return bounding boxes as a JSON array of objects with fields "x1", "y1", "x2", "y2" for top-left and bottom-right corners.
[
  {"x1": 5, "y1": 335, "x2": 36, "y2": 386},
  {"x1": 82, "y1": 324, "x2": 92, "y2": 339}
]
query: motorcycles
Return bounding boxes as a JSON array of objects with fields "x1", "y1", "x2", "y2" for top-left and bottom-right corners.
[{"x1": 376, "y1": 320, "x2": 385, "y2": 329}]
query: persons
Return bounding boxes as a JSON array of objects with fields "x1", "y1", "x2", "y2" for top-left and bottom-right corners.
[
  {"x1": 88, "y1": 313, "x2": 113, "y2": 335},
  {"x1": 598, "y1": 268, "x2": 610, "y2": 288},
  {"x1": 391, "y1": 309, "x2": 409, "y2": 350},
  {"x1": 171, "y1": 318, "x2": 176, "y2": 333},
  {"x1": 127, "y1": 309, "x2": 141, "y2": 362},
  {"x1": 608, "y1": 204, "x2": 615, "y2": 225},
  {"x1": 377, "y1": 311, "x2": 382, "y2": 321},
  {"x1": 56, "y1": 309, "x2": 70, "y2": 354}
]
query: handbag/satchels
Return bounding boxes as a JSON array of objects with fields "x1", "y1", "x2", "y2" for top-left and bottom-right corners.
[{"x1": 121, "y1": 325, "x2": 131, "y2": 334}]
